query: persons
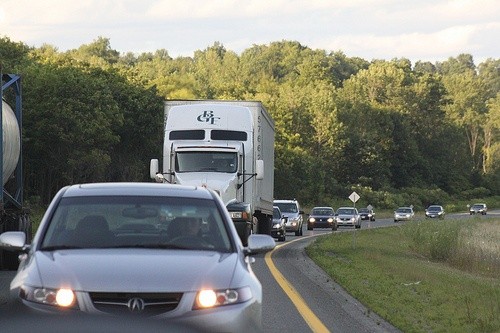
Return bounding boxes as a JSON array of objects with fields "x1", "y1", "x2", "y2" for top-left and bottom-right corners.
[
  {"x1": 164, "y1": 212, "x2": 207, "y2": 246},
  {"x1": 218, "y1": 158, "x2": 232, "y2": 169}
]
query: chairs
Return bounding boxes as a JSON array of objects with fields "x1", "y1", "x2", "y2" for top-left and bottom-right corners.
[{"x1": 70, "y1": 215, "x2": 159, "y2": 250}]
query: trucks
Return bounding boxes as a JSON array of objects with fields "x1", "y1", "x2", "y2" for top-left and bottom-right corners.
[{"x1": 149, "y1": 97, "x2": 276, "y2": 247}]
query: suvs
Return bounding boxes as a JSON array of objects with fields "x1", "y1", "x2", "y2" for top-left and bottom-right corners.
[{"x1": 274, "y1": 198, "x2": 305, "y2": 236}]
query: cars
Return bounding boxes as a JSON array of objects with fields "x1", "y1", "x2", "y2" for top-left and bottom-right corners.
[
  {"x1": 424, "y1": 205, "x2": 446, "y2": 220},
  {"x1": 273, "y1": 207, "x2": 289, "y2": 241},
  {"x1": 306, "y1": 206, "x2": 339, "y2": 231},
  {"x1": 394, "y1": 206, "x2": 415, "y2": 222},
  {"x1": 358, "y1": 208, "x2": 376, "y2": 222},
  {"x1": 469, "y1": 203, "x2": 488, "y2": 215},
  {"x1": 0, "y1": 181, "x2": 277, "y2": 333},
  {"x1": 335, "y1": 207, "x2": 363, "y2": 229}
]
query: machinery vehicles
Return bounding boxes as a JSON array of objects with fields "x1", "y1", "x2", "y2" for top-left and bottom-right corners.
[{"x1": 0, "y1": 72, "x2": 33, "y2": 270}]
